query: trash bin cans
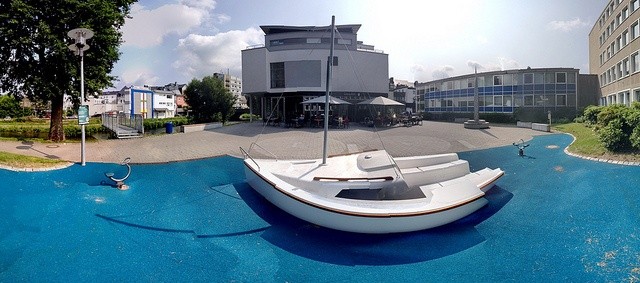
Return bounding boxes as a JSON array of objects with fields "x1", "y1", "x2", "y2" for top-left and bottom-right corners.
[{"x1": 165, "y1": 122, "x2": 172, "y2": 134}]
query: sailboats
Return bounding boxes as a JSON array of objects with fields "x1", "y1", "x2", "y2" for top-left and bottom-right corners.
[{"x1": 238, "y1": 15, "x2": 504, "y2": 234}]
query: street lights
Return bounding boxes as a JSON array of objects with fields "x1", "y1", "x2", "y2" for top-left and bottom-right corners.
[{"x1": 66, "y1": 27, "x2": 96, "y2": 165}]
